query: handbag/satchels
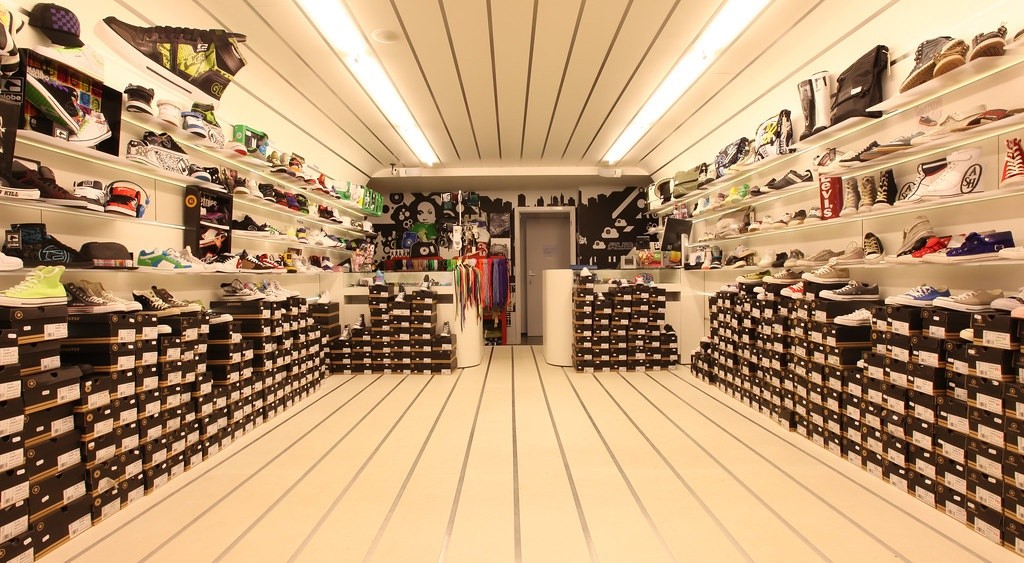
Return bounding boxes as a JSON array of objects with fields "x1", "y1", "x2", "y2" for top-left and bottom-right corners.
[{"x1": 830, "y1": 45, "x2": 891, "y2": 126}]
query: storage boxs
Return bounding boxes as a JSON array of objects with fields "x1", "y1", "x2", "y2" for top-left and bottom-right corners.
[
  {"x1": 0, "y1": 283, "x2": 457, "y2": 563},
  {"x1": 691, "y1": 283, "x2": 1024, "y2": 558},
  {"x1": 572, "y1": 273, "x2": 679, "y2": 373}
]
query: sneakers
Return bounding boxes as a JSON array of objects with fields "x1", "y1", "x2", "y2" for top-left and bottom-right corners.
[{"x1": 0, "y1": 0, "x2": 1024, "y2": 335}]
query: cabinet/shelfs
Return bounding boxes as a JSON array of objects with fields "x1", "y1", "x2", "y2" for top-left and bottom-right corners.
[
  {"x1": 648, "y1": 32, "x2": 1024, "y2": 272},
  {"x1": 0, "y1": 99, "x2": 368, "y2": 276}
]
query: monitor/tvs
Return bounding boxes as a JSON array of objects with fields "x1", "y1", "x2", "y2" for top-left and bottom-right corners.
[{"x1": 661, "y1": 216, "x2": 693, "y2": 268}]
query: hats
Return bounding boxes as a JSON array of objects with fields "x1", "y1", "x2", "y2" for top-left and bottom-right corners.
[{"x1": 27, "y1": 3, "x2": 84, "y2": 46}]
